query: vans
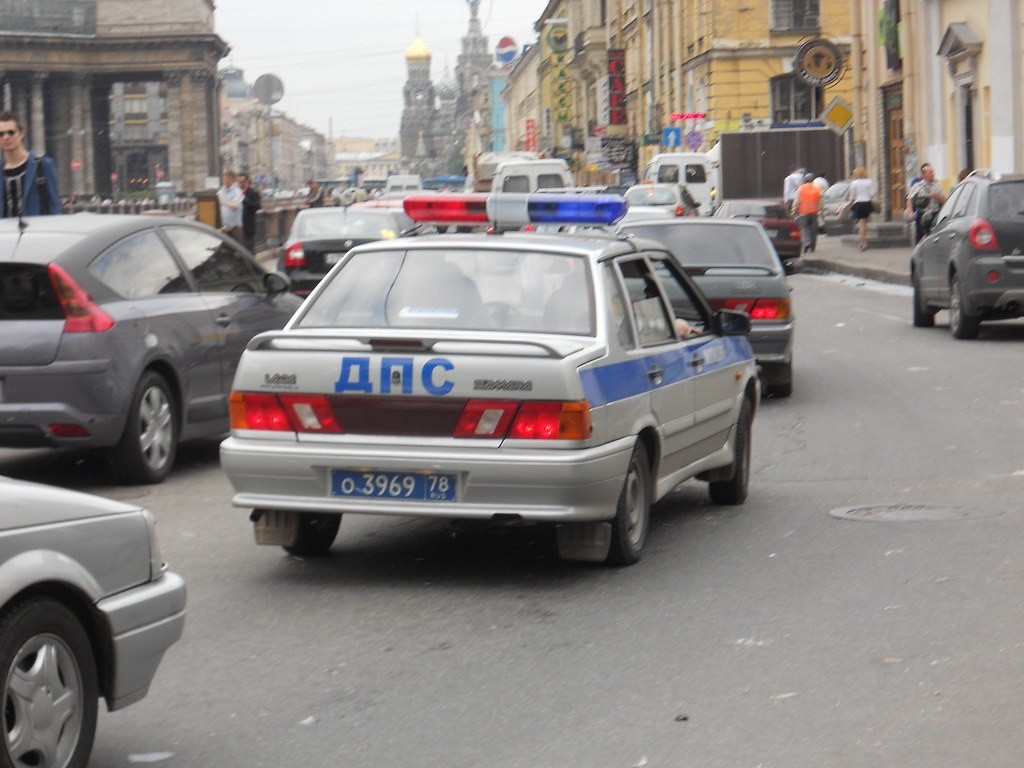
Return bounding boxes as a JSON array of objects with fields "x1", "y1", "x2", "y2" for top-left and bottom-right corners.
[
  {"x1": 641, "y1": 152, "x2": 716, "y2": 217},
  {"x1": 490, "y1": 159, "x2": 576, "y2": 235},
  {"x1": 386, "y1": 175, "x2": 422, "y2": 192}
]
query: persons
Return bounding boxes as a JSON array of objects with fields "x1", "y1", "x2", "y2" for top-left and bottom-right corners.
[
  {"x1": 791, "y1": 173, "x2": 821, "y2": 253},
  {"x1": 0, "y1": 112, "x2": 61, "y2": 218},
  {"x1": 906, "y1": 163, "x2": 970, "y2": 245},
  {"x1": 216, "y1": 171, "x2": 243, "y2": 243},
  {"x1": 306, "y1": 179, "x2": 324, "y2": 208},
  {"x1": 238, "y1": 173, "x2": 260, "y2": 256},
  {"x1": 849, "y1": 167, "x2": 872, "y2": 253},
  {"x1": 813, "y1": 172, "x2": 829, "y2": 193},
  {"x1": 783, "y1": 165, "x2": 804, "y2": 215}
]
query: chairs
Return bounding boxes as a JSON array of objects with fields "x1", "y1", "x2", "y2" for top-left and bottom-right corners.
[
  {"x1": 516, "y1": 259, "x2": 588, "y2": 337},
  {"x1": 398, "y1": 261, "x2": 503, "y2": 330}
]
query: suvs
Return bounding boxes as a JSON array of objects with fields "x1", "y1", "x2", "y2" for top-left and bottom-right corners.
[{"x1": 910, "y1": 168, "x2": 1024, "y2": 340}]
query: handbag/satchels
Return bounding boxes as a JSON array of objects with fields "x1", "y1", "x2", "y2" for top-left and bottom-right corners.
[{"x1": 902, "y1": 208, "x2": 919, "y2": 222}]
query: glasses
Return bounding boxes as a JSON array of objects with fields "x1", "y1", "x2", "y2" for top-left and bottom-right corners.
[{"x1": 0, "y1": 130, "x2": 20, "y2": 136}]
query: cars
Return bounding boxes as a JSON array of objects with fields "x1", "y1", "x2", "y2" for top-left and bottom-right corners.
[
  {"x1": 710, "y1": 199, "x2": 802, "y2": 263},
  {"x1": 0, "y1": 472, "x2": 186, "y2": 768},
  {"x1": 621, "y1": 183, "x2": 699, "y2": 221},
  {"x1": 276, "y1": 189, "x2": 438, "y2": 297},
  {"x1": 607, "y1": 217, "x2": 794, "y2": 402},
  {"x1": 220, "y1": 196, "x2": 763, "y2": 567},
  {"x1": 0, "y1": 213, "x2": 307, "y2": 487}
]
